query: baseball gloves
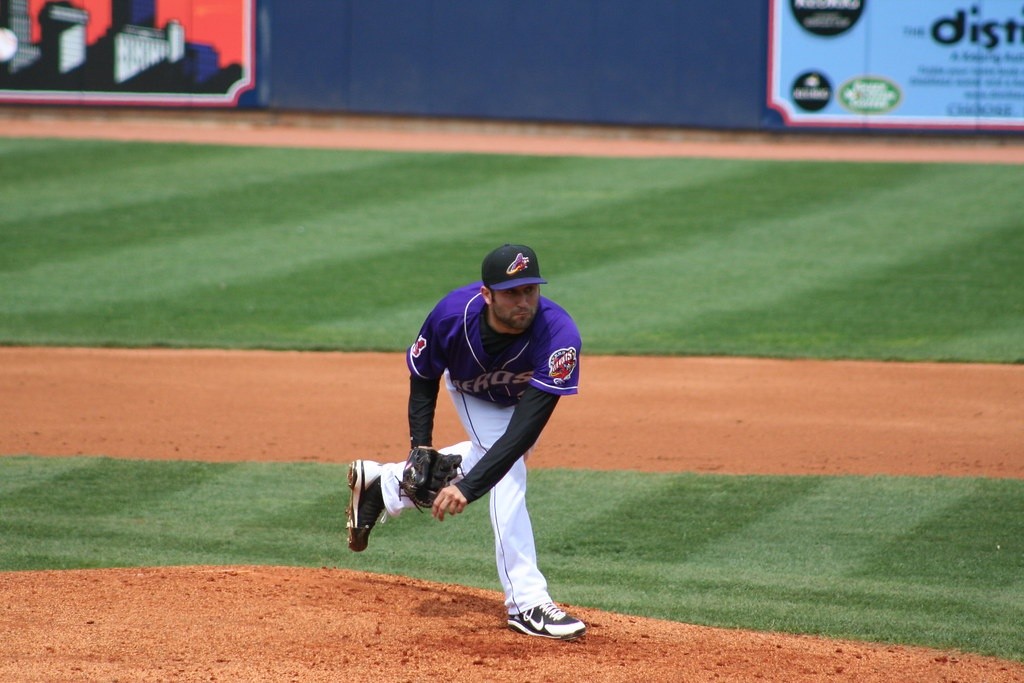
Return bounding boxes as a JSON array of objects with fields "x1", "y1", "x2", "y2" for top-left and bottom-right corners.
[{"x1": 402, "y1": 447, "x2": 463, "y2": 508}]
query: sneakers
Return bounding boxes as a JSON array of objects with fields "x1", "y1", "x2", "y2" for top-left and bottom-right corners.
[
  {"x1": 508, "y1": 601, "x2": 586, "y2": 640},
  {"x1": 347, "y1": 460, "x2": 385, "y2": 552}
]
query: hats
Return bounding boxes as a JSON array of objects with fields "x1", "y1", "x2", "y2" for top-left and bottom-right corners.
[{"x1": 482, "y1": 244, "x2": 548, "y2": 290}]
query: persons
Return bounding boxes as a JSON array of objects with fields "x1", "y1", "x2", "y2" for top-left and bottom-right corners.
[{"x1": 346, "y1": 244, "x2": 586, "y2": 640}]
